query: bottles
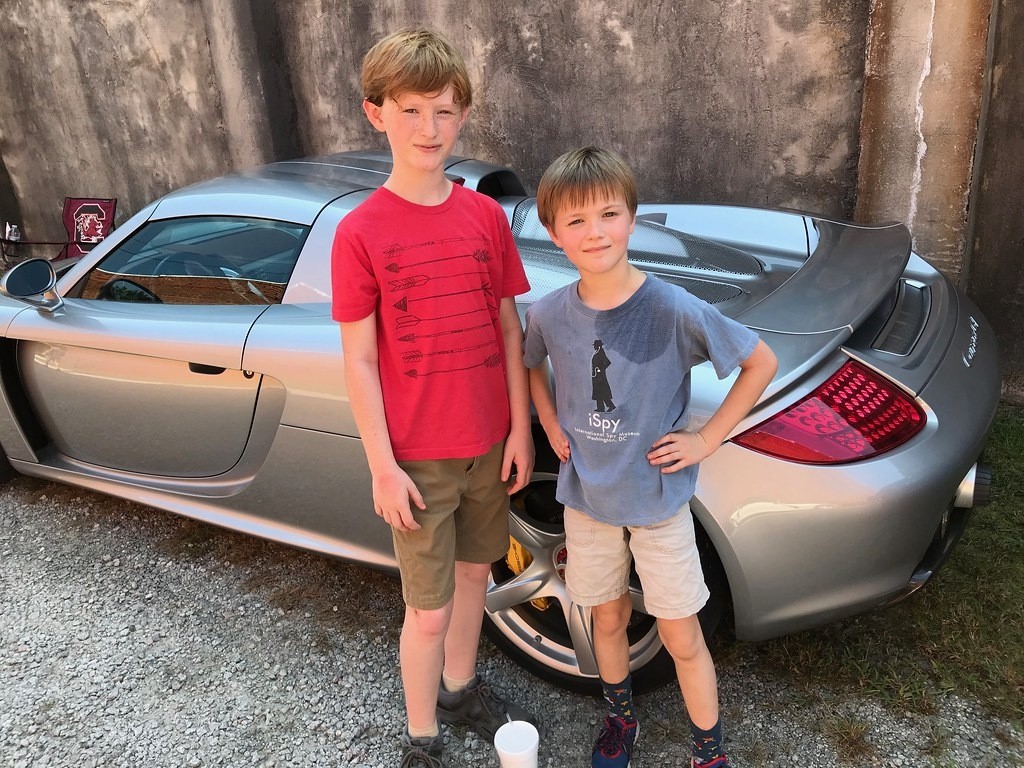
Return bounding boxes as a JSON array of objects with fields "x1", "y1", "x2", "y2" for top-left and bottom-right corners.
[{"x1": 6, "y1": 225, "x2": 20, "y2": 256}]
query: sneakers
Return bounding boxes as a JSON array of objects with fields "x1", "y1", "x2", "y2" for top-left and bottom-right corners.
[
  {"x1": 691, "y1": 753, "x2": 731, "y2": 768},
  {"x1": 435, "y1": 677, "x2": 537, "y2": 747},
  {"x1": 591, "y1": 714, "x2": 640, "y2": 768},
  {"x1": 402, "y1": 718, "x2": 443, "y2": 768}
]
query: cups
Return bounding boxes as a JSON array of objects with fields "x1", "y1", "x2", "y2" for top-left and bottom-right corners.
[{"x1": 494, "y1": 721, "x2": 539, "y2": 768}]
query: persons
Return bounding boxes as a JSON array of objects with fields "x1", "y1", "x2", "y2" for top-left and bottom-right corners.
[
  {"x1": 331, "y1": 26, "x2": 536, "y2": 768},
  {"x1": 519, "y1": 145, "x2": 778, "y2": 768}
]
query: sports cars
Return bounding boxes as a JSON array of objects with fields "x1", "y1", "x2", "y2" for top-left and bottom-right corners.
[{"x1": 1, "y1": 148, "x2": 1002, "y2": 699}]
